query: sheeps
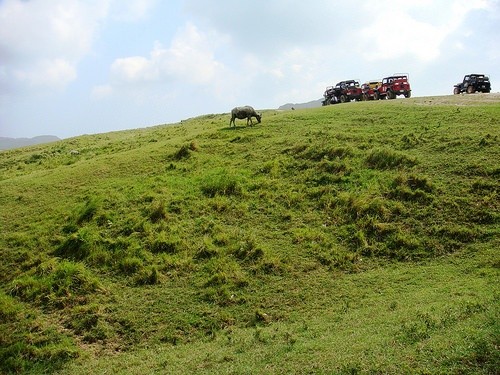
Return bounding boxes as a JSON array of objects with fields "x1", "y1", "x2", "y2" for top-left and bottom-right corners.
[{"x1": 229, "y1": 105, "x2": 262, "y2": 128}]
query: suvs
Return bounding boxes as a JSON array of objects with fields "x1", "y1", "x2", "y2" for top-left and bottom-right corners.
[
  {"x1": 326, "y1": 74, "x2": 410, "y2": 100},
  {"x1": 453, "y1": 73, "x2": 491, "y2": 95}
]
